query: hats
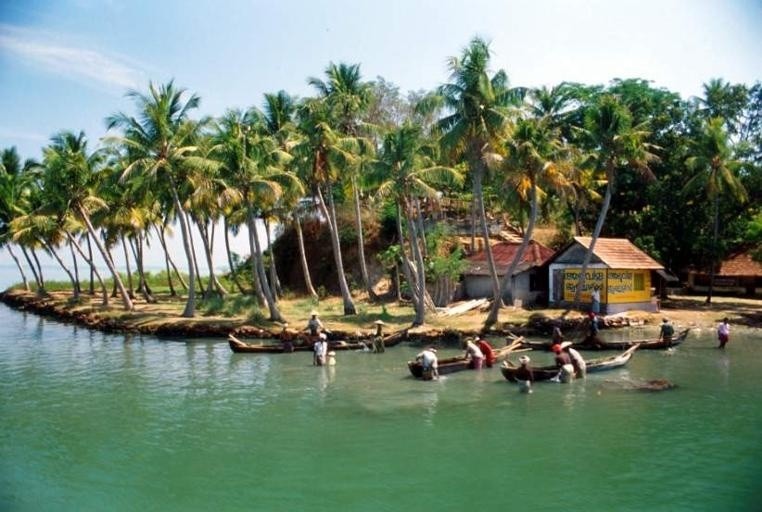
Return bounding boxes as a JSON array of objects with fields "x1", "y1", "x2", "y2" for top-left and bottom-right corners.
[
  {"x1": 559, "y1": 339, "x2": 573, "y2": 351},
  {"x1": 373, "y1": 319, "x2": 385, "y2": 325},
  {"x1": 309, "y1": 308, "x2": 320, "y2": 316}
]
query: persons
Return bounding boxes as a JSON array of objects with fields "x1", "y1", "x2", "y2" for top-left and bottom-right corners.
[
  {"x1": 588, "y1": 311, "x2": 599, "y2": 338},
  {"x1": 552, "y1": 319, "x2": 587, "y2": 382},
  {"x1": 474, "y1": 336, "x2": 496, "y2": 367},
  {"x1": 658, "y1": 318, "x2": 675, "y2": 348},
  {"x1": 514, "y1": 355, "x2": 534, "y2": 392},
  {"x1": 461, "y1": 337, "x2": 484, "y2": 369},
  {"x1": 280, "y1": 312, "x2": 338, "y2": 367},
  {"x1": 718, "y1": 318, "x2": 730, "y2": 349},
  {"x1": 416, "y1": 345, "x2": 440, "y2": 381},
  {"x1": 590, "y1": 284, "x2": 601, "y2": 312},
  {"x1": 374, "y1": 319, "x2": 386, "y2": 352}
]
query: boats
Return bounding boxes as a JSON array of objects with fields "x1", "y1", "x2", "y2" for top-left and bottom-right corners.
[
  {"x1": 499, "y1": 341, "x2": 642, "y2": 383},
  {"x1": 407, "y1": 336, "x2": 522, "y2": 379},
  {"x1": 504, "y1": 328, "x2": 691, "y2": 351},
  {"x1": 329, "y1": 326, "x2": 410, "y2": 349},
  {"x1": 226, "y1": 333, "x2": 314, "y2": 352}
]
